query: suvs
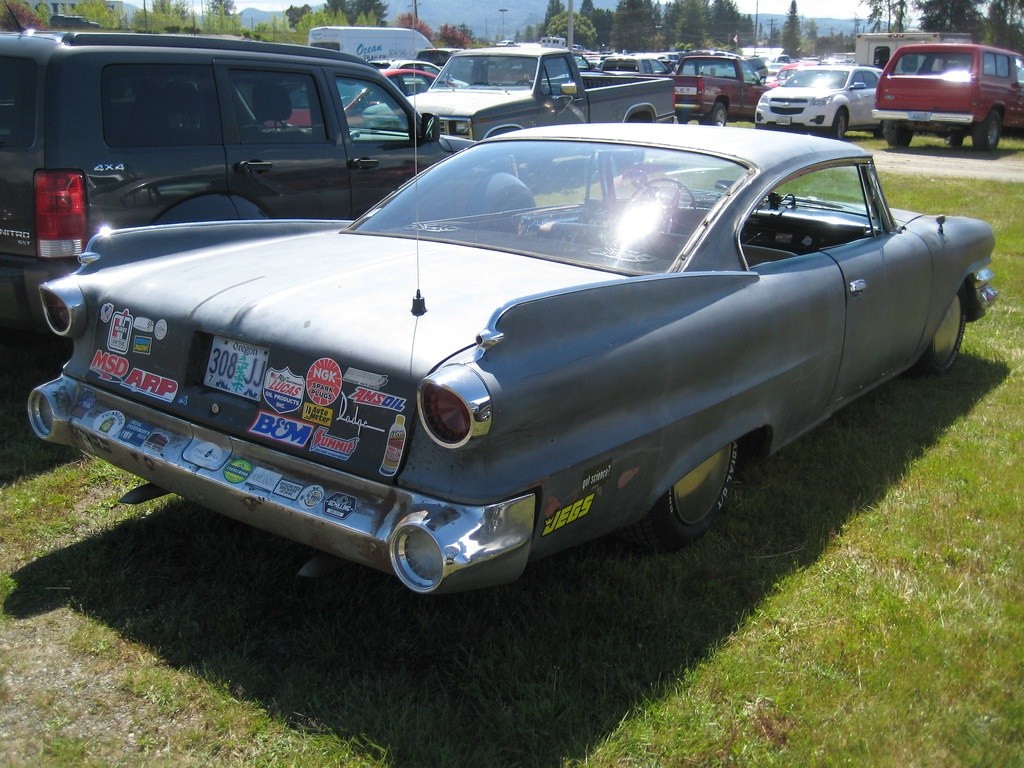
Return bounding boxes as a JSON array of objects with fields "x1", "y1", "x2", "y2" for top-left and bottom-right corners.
[
  {"x1": 600, "y1": 55, "x2": 674, "y2": 79},
  {"x1": 496, "y1": 41, "x2": 513, "y2": 46},
  {"x1": 0, "y1": 28, "x2": 520, "y2": 382},
  {"x1": 415, "y1": 46, "x2": 465, "y2": 69},
  {"x1": 871, "y1": 43, "x2": 1024, "y2": 152},
  {"x1": 51, "y1": 14, "x2": 100, "y2": 29}
]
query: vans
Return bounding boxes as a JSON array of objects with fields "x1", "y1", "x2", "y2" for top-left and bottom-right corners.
[{"x1": 308, "y1": 25, "x2": 433, "y2": 59}]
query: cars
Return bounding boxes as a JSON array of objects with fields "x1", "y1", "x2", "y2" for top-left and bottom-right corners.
[
  {"x1": 26, "y1": 121, "x2": 994, "y2": 600},
  {"x1": 571, "y1": 51, "x2": 607, "y2": 72},
  {"x1": 261, "y1": 70, "x2": 455, "y2": 133},
  {"x1": 765, "y1": 63, "x2": 816, "y2": 88},
  {"x1": 751, "y1": 67, "x2": 885, "y2": 140},
  {"x1": 368, "y1": 59, "x2": 469, "y2": 91},
  {"x1": 774, "y1": 52, "x2": 858, "y2": 69}
]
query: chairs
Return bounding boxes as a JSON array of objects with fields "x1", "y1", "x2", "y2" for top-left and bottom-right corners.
[
  {"x1": 245, "y1": 82, "x2": 307, "y2": 140},
  {"x1": 171, "y1": 78, "x2": 201, "y2": 134}
]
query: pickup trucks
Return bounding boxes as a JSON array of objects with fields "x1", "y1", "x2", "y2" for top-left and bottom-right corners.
[
  {"x1": 633, "y1": 56, "x2": 771, "y2": 129},
  {"x1": 363, "y1": 46, "x2": 676, "y2": 173}
]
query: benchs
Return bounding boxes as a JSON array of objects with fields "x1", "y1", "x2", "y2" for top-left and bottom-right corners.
[{"x1": 538, "y1": 220, "x2": 797, "y2": 264}]
